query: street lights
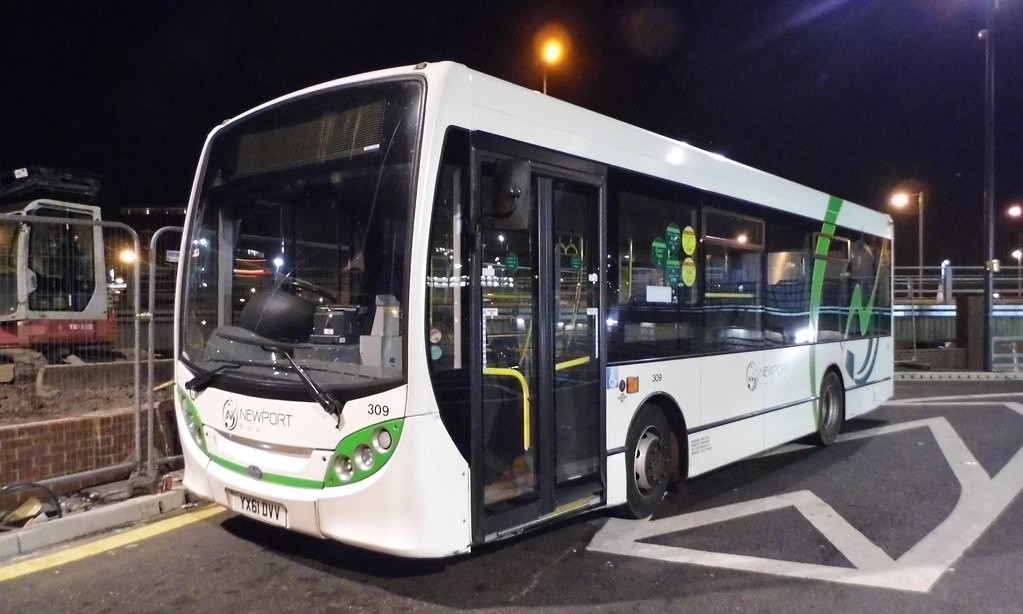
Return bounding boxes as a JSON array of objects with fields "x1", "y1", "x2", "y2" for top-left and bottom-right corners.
[
  {"x1": 892, "y1": 189, "x2": 925, "y2": 298},
  {"x1": 539, "y1": 38, "x2": 564, "y2": 96}
]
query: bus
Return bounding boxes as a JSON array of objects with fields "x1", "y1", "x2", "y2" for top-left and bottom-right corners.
[{"x1": 173, "y1": 61, "x2": 893, "y2": 560}]
